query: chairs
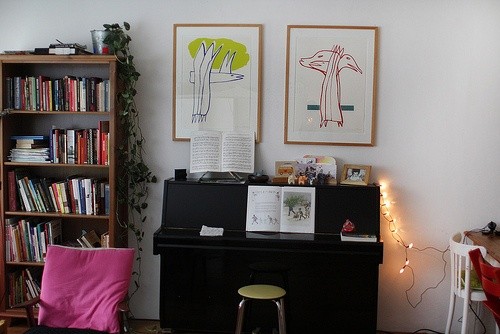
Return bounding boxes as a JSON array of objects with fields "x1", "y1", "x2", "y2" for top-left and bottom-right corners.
[
  {"x1": 443, "y1": 232, "x2": 500, "y2": 334},
  {"x1": 11, "y1": 244, "x2": 136, "y2": 334}
]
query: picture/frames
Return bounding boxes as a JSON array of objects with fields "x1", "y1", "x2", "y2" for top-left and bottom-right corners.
[
  {"x1": 284, "y1": 25, "x2": 378, "y2": 147},
  {"x1": 339, "y1": 164, "x2": 371, "y2": 186},
  {"x1": 171, "y1": 23, "x2": 263, "y2": 143}
]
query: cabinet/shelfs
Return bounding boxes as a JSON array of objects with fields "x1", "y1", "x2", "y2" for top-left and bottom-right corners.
[{"x1": 0, "y1": 54, "x2": 127, "y2": 326}]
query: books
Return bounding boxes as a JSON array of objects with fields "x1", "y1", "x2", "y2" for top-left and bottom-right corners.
[
  {"x1": 8, "y1": 267, "x2": 40, "y2": 307},
  {"x1": 5, "y1": 75, "x2": 110, "y2": 112},
  {"x1": 190, "y1": 130, "x2": 255, "y2": 173},
  {"x1": 5, "y1": 218, "x2": 109, "y2": 262},
  {"x1": 246, "y1": 185, "x2": 316, "y2": 234},
  {"x1": 7, "y1": 170, "x2": 110, "y2": 215},
  {"x1": 7, "y1": 120, "x2": 110, "y2": 166},
  {"x1": 341, "y1": 232, "x2": 377, "y2": 242}
]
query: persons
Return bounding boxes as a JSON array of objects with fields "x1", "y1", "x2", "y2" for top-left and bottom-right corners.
[
  {"x1": 306, "y1": 164, "x2": 316, "y2": 172},
  {"x1": 288, "y1": 203, "x2": 295, "y2": 216},
  {"x1": 297, "y1": 205, "x2": 310, "y2": 220},
  {"x1": 350, "y1": 171, "x2": 362, "y2": 181},
  {"x1": 317, "y1": 168, "x2": 331, "y2": 179}
]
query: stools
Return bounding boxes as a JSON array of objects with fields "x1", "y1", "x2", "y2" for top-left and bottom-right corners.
[{"x1": 236, "y1": 284, "x2": 286, "y2": 334}]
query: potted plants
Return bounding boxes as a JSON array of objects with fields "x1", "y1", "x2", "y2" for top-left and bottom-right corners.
[{"x1": 90, "y1": 21, "x2": 157, "y2": 304}]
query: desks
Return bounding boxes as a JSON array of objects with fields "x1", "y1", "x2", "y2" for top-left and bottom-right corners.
[{"x1": 464, "y1": 231, "x2": 500, "y2": 268}]
